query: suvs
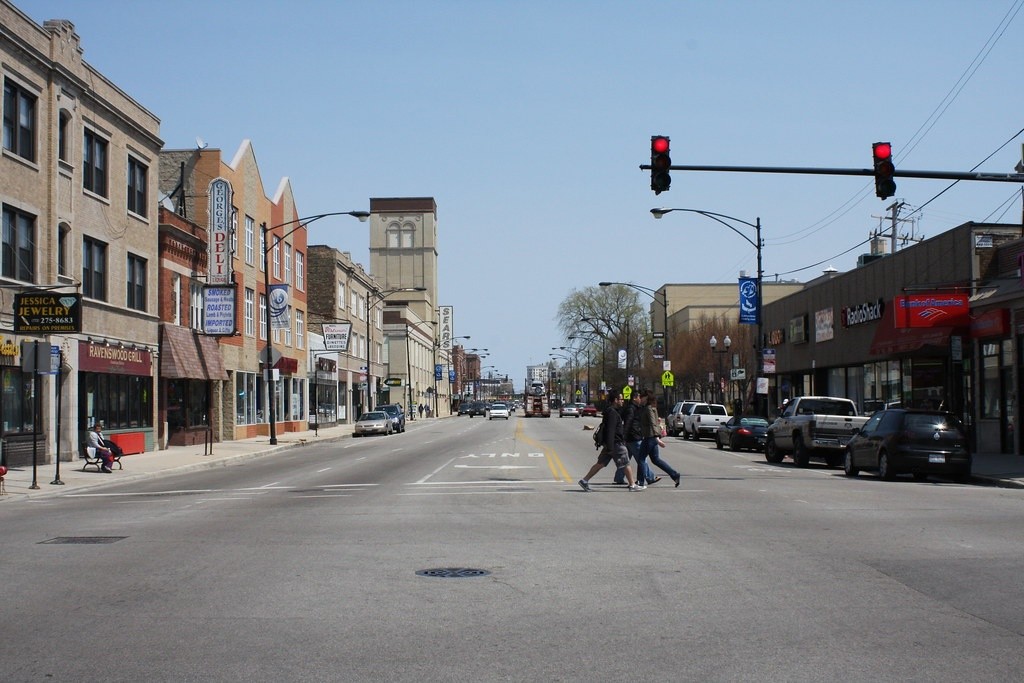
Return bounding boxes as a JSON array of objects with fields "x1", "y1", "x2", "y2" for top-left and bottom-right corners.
[
  {"x1": 373, "y1": 403, "x2": 406, "y2": 433},
  {"x1": 528, "y1": 380, "x2": 547, "y2": 397},
  {"x1": 666, "y1": 399, "x2": 713, "y2": 437}
]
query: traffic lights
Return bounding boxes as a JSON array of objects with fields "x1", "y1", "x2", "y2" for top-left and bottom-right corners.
[
  {"x1": 872, "y1": 141, "x2": 897, "y2": 200},
  {"x1": 651, "y1": 134, "x2": 672, "y2": 196}
]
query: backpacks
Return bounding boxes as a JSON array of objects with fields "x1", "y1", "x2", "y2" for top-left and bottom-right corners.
[{"x1": 593, "y1": 422, "x2": 606, "y2": 447}]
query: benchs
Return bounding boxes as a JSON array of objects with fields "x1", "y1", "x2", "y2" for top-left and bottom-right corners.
[{"x1": 82, "y1": 440, "x2": 122, "y2": 473}]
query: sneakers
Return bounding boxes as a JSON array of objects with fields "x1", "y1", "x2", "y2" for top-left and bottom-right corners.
[
  {"x1": 629, "y1": 484, "x2": 647, "y2": 492},
  {"x1": 578, "y1": 480, "x2": 592, "y2": 491}
]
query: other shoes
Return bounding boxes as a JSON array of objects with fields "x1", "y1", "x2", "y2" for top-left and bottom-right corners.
[
  {"x1": 101, "y1": 465, "x2": 112, "y2": 473},
  {"x1": 674, "y1": 472, "x2": 680, "y2": 487},
  {"x1": 648, "y1": 477, "x2": 661, "y2": 485},
  {"x1": 612, "y1": 479, "x2": 628, "y2": 487}
]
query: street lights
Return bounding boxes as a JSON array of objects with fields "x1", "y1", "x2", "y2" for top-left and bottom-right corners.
[
  {"x1": 649, "y1": 207, "x2": 765, "y2": 419},
  {"x1": 567, "y1": 336, "x2": 606, "y2": 381},
  {"x1": 459, "y1": 348, "x2": 488, "y2": 403},
  {"x1": 552, "y1": 347, "x2": 579, "y2": 402},
  {"x1": 405, "y1": 321, "x2": 438, "y2": 421},
  {"x1": 488, "y1": 370, "x2": 499, "y2": 400},
  {"x1": 262, "y1": 210, "x2": 372, "y2": 446},
  {"x1": 710, "y1": 334, "x2": 733, "y2": 405},
  {"x1": 474, "y1": 365, "x2": 494, "y2": 402},
  {"x1": 366, "y1": 287, "x2": 427, "y2": 411},
  {"x1": 432, "y1": 335, "x2": 471, "y2": 417},
  {"x1": 599, "y1": 281, "x2": 671, "y2": 418},
  {"x1": 560, "y1": 347, "x2": 590, "y2": 404},
  {"x1": 580, "y1": 317, "x2": 629, "y2": 386},
  {"x1": 548, "y1": 354, "x2": 573, "y2": 403},
  {"x1": 447, "y1": 346, "x2": 478, "y2": 415}
]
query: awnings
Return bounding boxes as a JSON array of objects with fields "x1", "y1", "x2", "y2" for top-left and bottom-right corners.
[
  {"x1": 869, "y1": 300, "x2": 971, "y2": 356},
  {"x1": 161, "y1": 323, "x2": 230, "y2": 381}
]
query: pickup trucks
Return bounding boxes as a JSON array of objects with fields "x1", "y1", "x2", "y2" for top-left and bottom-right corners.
[
  {"x1": 683, "y1": 403, "x2": 734, "y2": 441},
  {"x1": 765, "y1": 395, "x2": 882, "y2": 468},
  {"x1": 861, "y1": 398, "x2": 902, "y2": 417}
]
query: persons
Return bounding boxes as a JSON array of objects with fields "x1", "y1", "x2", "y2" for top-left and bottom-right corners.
[
  {"x1": 634, "y1": 389, "x2": 681, "y2": 487},
  {"x1": 578, "y1": 391, "x2": 647, "y2": 492},
  {"x1": 86, "y1": 423, "x2": 113, "y2": 473},
  {"x1": 419, "y1": 404, "x2": 424, "y2": 418},
  {"x1": 424, "y1": 403, "x2": 430, "y2": 418},
  {"x1": 613, "y1": 393, "x2": 661, "y2": 486}
]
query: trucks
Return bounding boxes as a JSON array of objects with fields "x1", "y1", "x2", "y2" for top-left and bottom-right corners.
[{"x1": 523, "y1": 393, "x2": 551, "y2": 419}]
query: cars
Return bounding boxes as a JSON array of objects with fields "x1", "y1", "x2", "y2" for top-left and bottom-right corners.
[
  {"x1": 559, "y1": 403, "x2": 580, "y2": 418},
  {"x1": 548, "y1": 398, "x2": 586, "y2": 415},
  {"x1": 471, "y1": 398, "x2": 525, "y2": 413},
  {"x1": 580, "y1": 403, "x2": 598, "y2": 417},
  {"x1": 457, "y1": 403, "x2": 473, "y2": 416},
  {"x1": 355, "y1": 410, "x2": 393, "y2": 436},
  {"x1": 715, "y1": 414, "x2": 771, "y2": 453},
  {"x1": 843, "y1": 406, "x2": 974, "y2": 485},
  {"x1": 488, "y1": 403, "x2": 510, "y2": 420}
]
